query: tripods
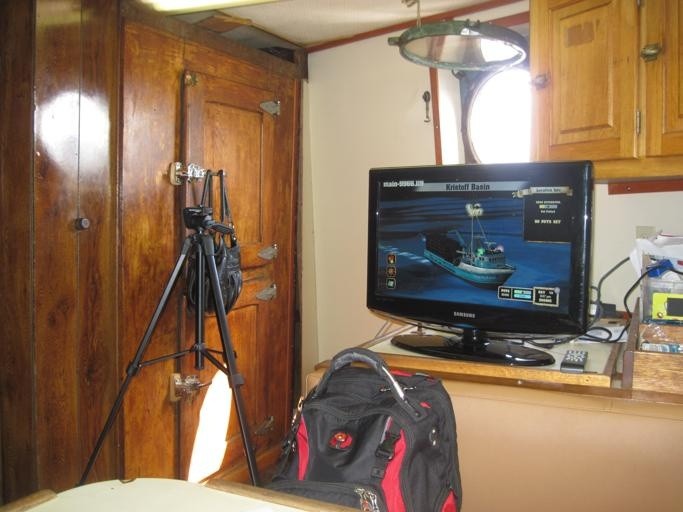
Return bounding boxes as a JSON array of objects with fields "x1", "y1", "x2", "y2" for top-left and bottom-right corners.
[{"x1": 78, "y1": 230, "x2": 260, "y2": 485}]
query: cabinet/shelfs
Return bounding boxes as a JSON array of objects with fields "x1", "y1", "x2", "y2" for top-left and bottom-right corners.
[
  {"x1": 643, "y1": 0, "x2": 683, "y2": 178},
  {"x1": 0, "y1": 1, "x2": 304, "y2": 512},
  {"x1": 530, "y1": 0, "x2": 644, "y2": 182}
]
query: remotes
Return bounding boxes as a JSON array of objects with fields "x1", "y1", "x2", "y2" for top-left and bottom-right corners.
[{"x1": 560, "y1": 348, "x2": 588, "y2": 371}]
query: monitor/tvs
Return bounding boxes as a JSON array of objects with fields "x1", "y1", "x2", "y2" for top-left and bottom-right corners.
[{"x1": 365, "y1": 160, "x2": 593, "y2": 366}]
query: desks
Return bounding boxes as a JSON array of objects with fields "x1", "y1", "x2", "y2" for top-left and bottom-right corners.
[
  {"x1": 0, "y1": 476, "x2": 371, "y2": 512},
  {"x1": 304, "y1": 315, "x2": 683, "y2": 512}
]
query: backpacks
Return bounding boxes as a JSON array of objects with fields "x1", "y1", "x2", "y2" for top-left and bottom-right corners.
[{"x1": 262, "y1": 347, "x2": 462, "y2": 511}]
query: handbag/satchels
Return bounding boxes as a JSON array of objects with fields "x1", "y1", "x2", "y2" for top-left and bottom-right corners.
[{"x1": 185, "y1": 243, "x2": 242, "y2": 316}]
